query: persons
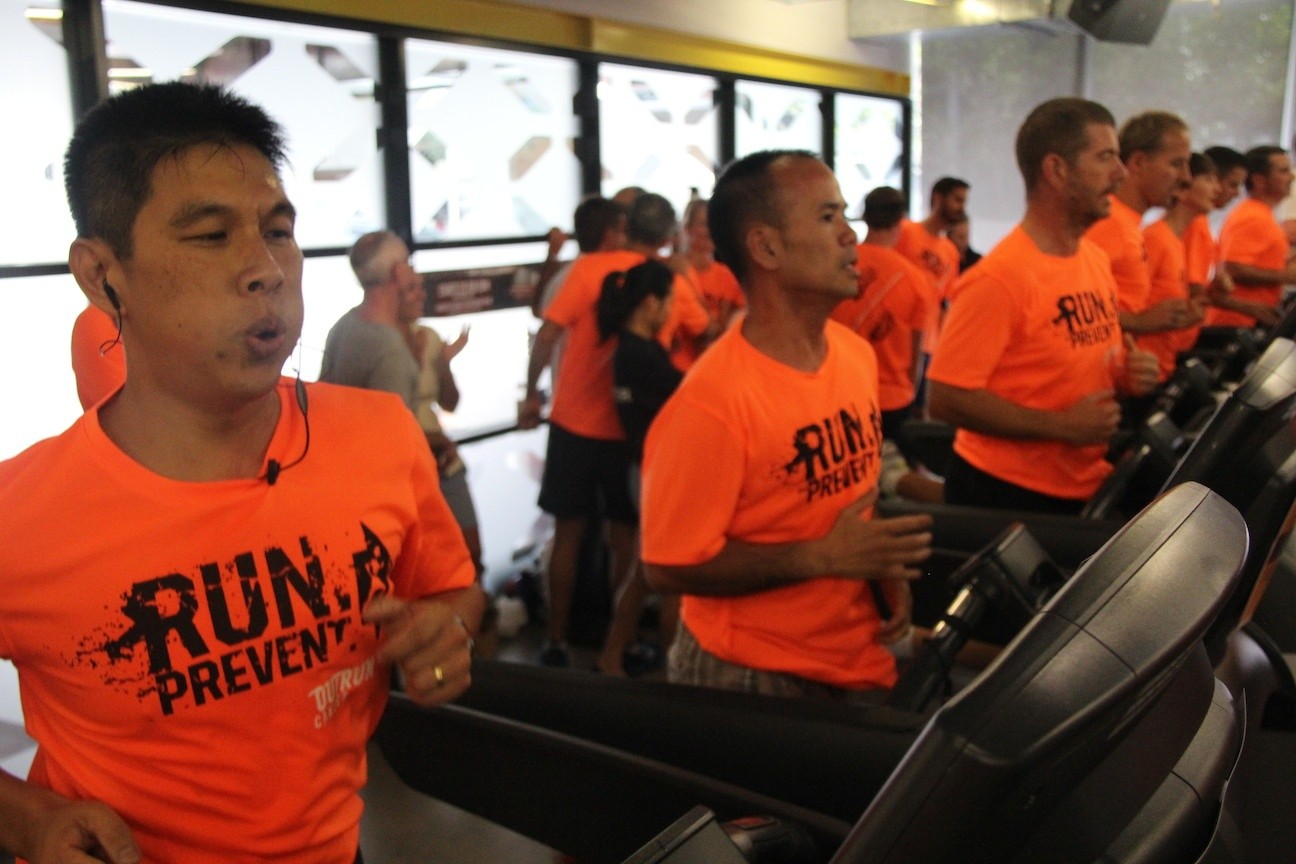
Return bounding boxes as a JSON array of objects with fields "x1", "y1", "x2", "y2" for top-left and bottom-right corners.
[
  {"x1": 1208, "y1": 145, "x2": 1296, "y2": 329},
  {"x1": 635, "y1": 149, "x2": 932, "y2": 711},
  {"x1": 1181, "y1": 147, "x2": 1296, "y2": 330},
  {"x1": 0, "y1": 78, "x2": 486, "y2": 862},
  {"x1": 396, "y1": 273, "x2": 485, "y2": 588},
  {"x1": 921, "y1": 96, "x2": 1168, "y2": 589},
  {"x1": 73, "y1": 298, "x2": 124, "y2": 416},
  {"x1": 1130, "y1": 152, "x2": 1222, "y2": 393},
  {"x1": 319, "y1": 227, "x2": 422, "y2": 420},
  {"x1": 1081, "y1": 111, "x2": 1206, "y2": 453},
  {"x1": 514, "y1": 175, "x2": 986, "y2": 694}
]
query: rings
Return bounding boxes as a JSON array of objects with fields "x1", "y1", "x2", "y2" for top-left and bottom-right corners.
[{"x1": 433, "y1": 664, "x2": 448, "y2": 690}]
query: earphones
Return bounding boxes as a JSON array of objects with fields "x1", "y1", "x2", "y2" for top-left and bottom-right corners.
[{"x1": 102, "y1": 277, "x2": 120, "y2": 311}]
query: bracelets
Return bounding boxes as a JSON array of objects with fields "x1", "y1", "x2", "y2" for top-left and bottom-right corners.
[{"x1": 451, "y1": 614, "x2": 477, "y2": 651}]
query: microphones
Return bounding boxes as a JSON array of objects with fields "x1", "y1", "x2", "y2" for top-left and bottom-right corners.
[{"x1": 295, "y1": 377, "x2": 310, "y2": 416}]
